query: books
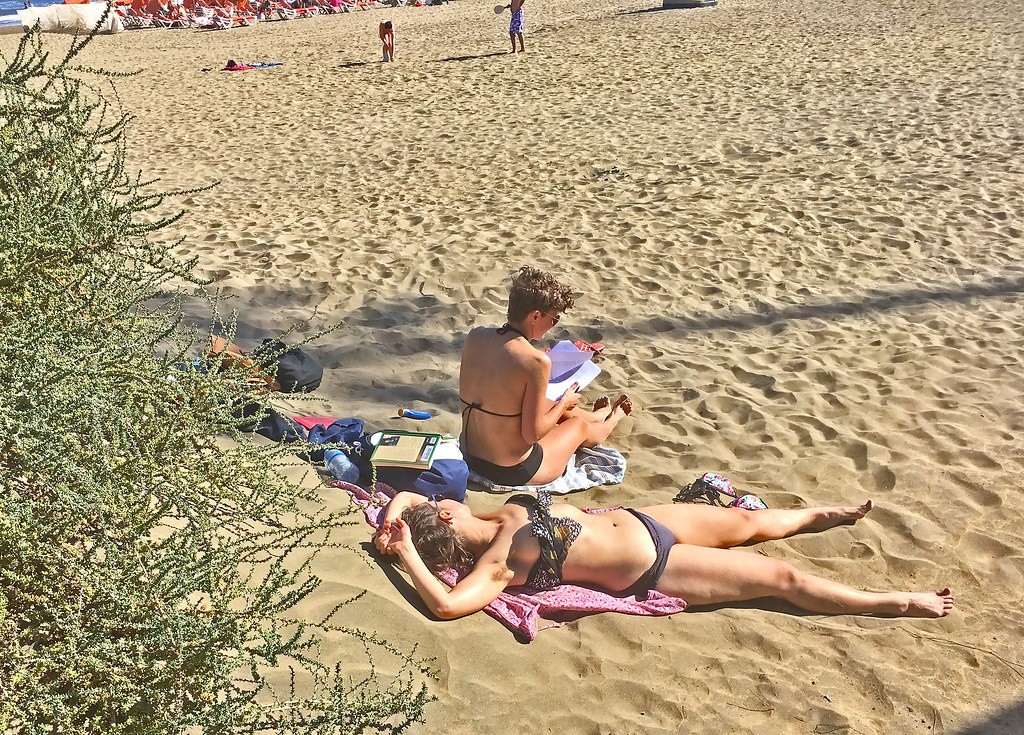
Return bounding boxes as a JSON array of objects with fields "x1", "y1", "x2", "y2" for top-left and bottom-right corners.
[{"x1": 370, "y1": 431, "x2": 442, "y2": 469}]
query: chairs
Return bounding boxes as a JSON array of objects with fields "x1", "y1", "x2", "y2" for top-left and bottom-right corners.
[{"x1": 112, "y1": 0, "x2": 421, "y2": 30}]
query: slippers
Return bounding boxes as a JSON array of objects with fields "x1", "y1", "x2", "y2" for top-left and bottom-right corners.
[{"x1": 573, "y1": 340, "x2": 605, "y2": 356}]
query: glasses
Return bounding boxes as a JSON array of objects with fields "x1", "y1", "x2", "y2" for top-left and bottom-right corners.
[
  {"x1": 429, "y1": 494, "x2": 453, "y2": 526},
  {"x1": 529, "y1": 308, "x2": 560, "y2": 327}
]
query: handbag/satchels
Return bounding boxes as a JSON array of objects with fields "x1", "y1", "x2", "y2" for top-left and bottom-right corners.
[{"x1": 205, "y1": 335, "x2": 282, "y2": 391}]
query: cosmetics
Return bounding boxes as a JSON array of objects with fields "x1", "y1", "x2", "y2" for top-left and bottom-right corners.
[{"x1": 398, "y1": 409, "x2": 430, "y2": 420}]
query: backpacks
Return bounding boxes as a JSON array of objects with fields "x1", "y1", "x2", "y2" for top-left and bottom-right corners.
[{"x1": 251, "y1": 339, "x2": 323, "y2": 392}]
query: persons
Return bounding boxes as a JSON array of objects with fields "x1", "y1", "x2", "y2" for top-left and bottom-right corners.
[
  {"x1": 505, "y1": 0, "x2": 525, "y2": 53},
  {"x1": 24, "y1": 1, "x2": 32, "y2": 9},
  {"x1": 376, "y1": 491, "x2": 954, "y2": 618},
  {"x1": 459, "y1": 271, "x2": 632, "y2": 485},
  {"x1": 116, "y1": 0, "x2": 422, "y2": 28},
  {"x1": 379, "y1": 20, "x2": 395, "y2": 62}
]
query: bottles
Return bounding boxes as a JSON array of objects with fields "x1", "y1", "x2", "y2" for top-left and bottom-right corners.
[
  {"x1": 398, "y1": 408, "x2": 432, "y2": 420},
  {"x1": 324, "y1": 449, "x2": 359, "y2": 484}
]
